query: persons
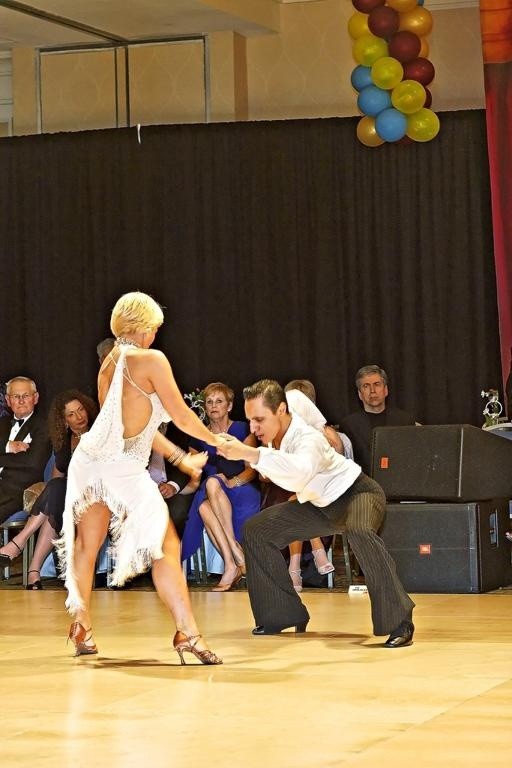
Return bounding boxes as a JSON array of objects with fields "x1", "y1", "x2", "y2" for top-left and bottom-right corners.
[
  {"x1": 97, "y1": 339, "x2": 116, "y2": 365},
  {"x1": 216, "y1": 378, "x2": 416, "y2": 647},
  {"x1": 49, "y1": 292, "x2": 227, "y2": 665},
  {"x1": 339, "y1": 364, "x2": 421, "y2": 477},
  {"x1": 1, "y1": 374, "x2": 344, "y2": 593}
]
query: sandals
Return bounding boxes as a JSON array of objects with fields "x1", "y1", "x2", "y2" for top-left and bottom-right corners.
[
  {"x1": 289, "y1": 568, "x2": 304, "y2": 591},
  {"x1": 27, "y1": 569, "x2": 44, "y2": 590},
  {"x1": 0, "y1": 540, "x2": 24, "y2": 564},
  {"x1": 311, "y1": 548, "x2": 336, "y2": 575}
]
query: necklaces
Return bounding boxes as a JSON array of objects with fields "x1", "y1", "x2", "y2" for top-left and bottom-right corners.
[{"x1": 114, "y1": 337, "x2": 142, "y2": 349}]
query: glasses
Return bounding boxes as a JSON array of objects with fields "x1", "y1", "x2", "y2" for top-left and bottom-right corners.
[{"x1": 13, "y1": 393, "x2": 31, "y2": 400}]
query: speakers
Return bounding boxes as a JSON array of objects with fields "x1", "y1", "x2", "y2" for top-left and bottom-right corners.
[
  {"x1": 378, "y1": 499, "x2": 512, "y2": 596},
  {"x1": 370, "y1": 424, "x2": 512, "y2": 502}
]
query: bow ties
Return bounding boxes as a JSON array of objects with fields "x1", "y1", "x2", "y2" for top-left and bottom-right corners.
[{"x1": 10, "y1": 418, "x2": 24, "y2": 426}]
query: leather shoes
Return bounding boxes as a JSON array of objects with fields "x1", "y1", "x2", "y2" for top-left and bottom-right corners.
[
  {"x1": 251, "y1": 619, "x2": 308, "y2": 635},
  {"x1": 386, "y1": 626, "x2": 415, "y2": 646},
  {"x1": 213, "y1": 540, "x2": 247, "y2": 592}
]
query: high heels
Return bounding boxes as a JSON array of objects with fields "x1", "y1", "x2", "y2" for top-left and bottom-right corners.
[
  {"x1": 68, "y1": 621, "x2": 98, "y2": 655},
  {"x1": 173, "y1": 631, "x2": 223, "y2": 664}
]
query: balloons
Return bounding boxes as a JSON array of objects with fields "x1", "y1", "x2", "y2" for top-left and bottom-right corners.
[{"x1": 348, "y1": 1, "x2": 440, "y2": 147}]
